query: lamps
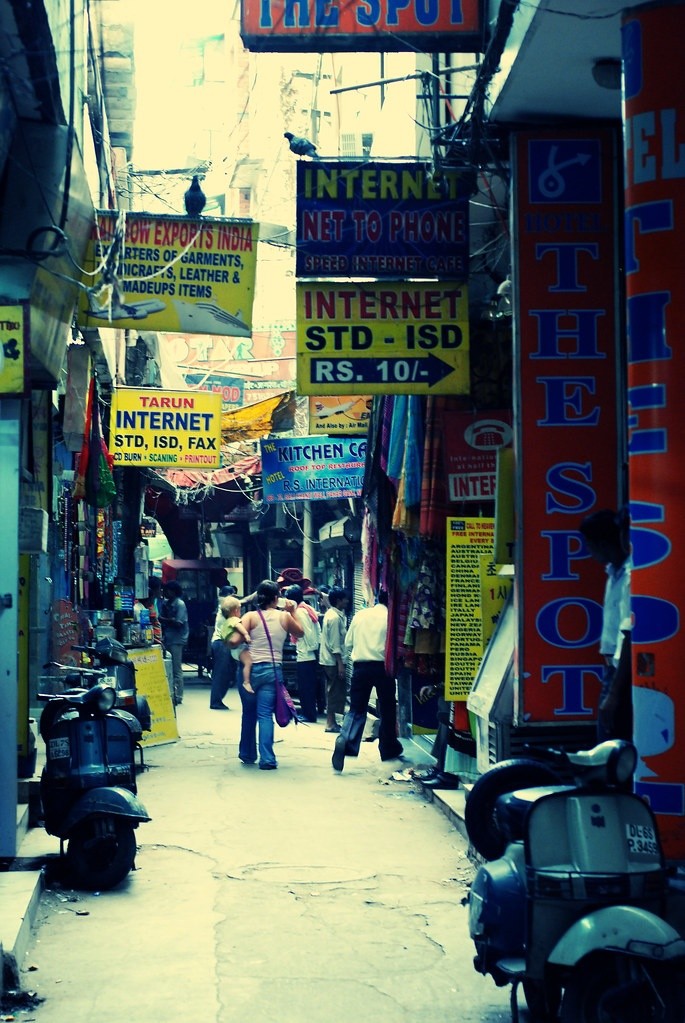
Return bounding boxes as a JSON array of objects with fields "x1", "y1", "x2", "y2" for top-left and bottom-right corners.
[{"x1": 591, "y1": 58, "x2": 622, "y2": 90}]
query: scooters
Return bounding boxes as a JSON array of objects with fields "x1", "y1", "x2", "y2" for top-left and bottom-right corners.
[
  {"x1": 464, "y1": 738, "x2": 685, "y2": 1023},
  {"x1": 36, "y1": 682, "x2": 152, "y2": 894},
  {"x1": 69, "y1": 638, "x2": 152, "y2": 745}
]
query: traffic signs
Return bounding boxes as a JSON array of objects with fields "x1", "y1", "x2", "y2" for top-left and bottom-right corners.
[{"x1": 295, "y1": 279, "x2": 471, "y2": 394}]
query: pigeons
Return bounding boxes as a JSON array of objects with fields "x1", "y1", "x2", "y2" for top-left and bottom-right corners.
[
  {"x1": 284, "y1": 132, "x2": 321, "y2": 162},
  {"x1": 185, "y1": 175, "x2": 207, "y2": 216}
]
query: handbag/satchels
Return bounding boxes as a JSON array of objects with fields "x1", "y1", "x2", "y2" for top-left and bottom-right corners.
[{"x1": 275, "y1": 681, "x2": 301, "y2": 727}]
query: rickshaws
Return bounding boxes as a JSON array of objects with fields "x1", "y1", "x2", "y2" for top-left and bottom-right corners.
[{"x1": 161, "y1": 557, "x2": 240, "y2": 689}]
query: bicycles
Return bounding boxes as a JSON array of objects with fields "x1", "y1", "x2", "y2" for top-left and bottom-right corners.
[{"x1": 42, "y1": 661, "x2": 108, "y2": 689}]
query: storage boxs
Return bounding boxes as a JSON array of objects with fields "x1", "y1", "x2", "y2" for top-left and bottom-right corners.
[{"x1": 465, "y1": 757, "x2": 560, "y2": 864}]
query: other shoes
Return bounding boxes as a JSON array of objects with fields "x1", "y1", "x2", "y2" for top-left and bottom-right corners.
[
  {"x1": 210, "y1": 701, "x2": 228, "y2": 710},
  {"x1": 331, "y1": 735, "x2": 348, "y2": 775}
]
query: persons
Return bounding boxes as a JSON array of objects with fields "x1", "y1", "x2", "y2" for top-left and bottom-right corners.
[
  {"x1": 146, "y1": 569, "x2": 404, "y2": 772},
  {"x1": 578, "y1": 510, "x2": 634, "y2": 742},
  {"x1": 414, "y1": 681, "x2": 460, "y2": 790}
]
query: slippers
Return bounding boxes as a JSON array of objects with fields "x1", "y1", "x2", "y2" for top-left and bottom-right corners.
[{"x1": 325, "y1": 724, "x2": 342, "y2": 732}]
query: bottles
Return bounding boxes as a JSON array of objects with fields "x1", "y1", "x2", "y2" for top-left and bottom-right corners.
[{"x1": 139, "y1": 607, "x2": 162, "y2": 644}]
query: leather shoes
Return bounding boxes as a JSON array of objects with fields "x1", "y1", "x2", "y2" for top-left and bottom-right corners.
[
  {"x1": 413, "y1": 767, "x2": 440, "y2": 781},
  {"x1": 420, "y1": 774, "x2": 460, "y2": 789}
]
